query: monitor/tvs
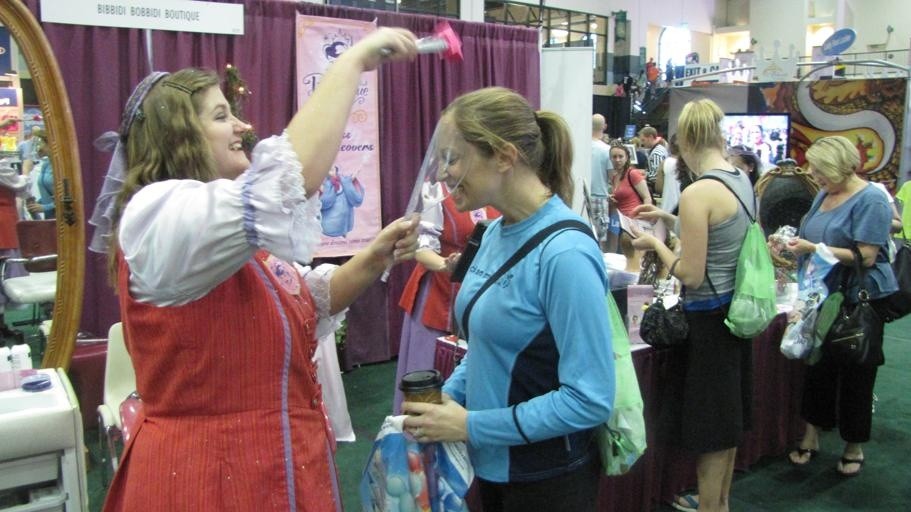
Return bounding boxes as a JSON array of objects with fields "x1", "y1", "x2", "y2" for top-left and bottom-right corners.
[{"x1": 718, "y1": 112, "x2": 792, "y2": 165}]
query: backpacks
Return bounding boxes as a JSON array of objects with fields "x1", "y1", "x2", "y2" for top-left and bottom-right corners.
[
  {"x1": 690, "y1": 174, "x2": 779, "y2": 339},
  {"x1": 539, "y1": 228, "x2": 650, "y2": 478}
]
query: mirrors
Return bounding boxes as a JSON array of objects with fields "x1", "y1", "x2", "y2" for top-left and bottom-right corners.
[{"x1": 0, "y1": 0, "x2": 84, "y2": 370}]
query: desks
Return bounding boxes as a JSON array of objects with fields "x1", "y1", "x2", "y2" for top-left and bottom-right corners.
[{"x1": 438, "y1": 282, "x2": 845, "y2": 512}]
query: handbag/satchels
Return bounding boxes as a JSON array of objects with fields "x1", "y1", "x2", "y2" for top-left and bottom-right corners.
[
  {"x1": 639, "y1": 257, "x2": 689, "y2": 349},
  {"x1": 878, "y1": 216, "x2": 911, "y2": 326},
  {"x1": 827, "y1": 243, "x2": 887, "y2": 363}
]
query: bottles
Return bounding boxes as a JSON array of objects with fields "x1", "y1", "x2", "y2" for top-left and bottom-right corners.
[
  {"x1": 10, "y1": 329, "x2": 35, "y2": 390},
  {"x1": 647, "y1": 62, "x2": 658, "y2": 81},
  {"x1": 1, "y1": 331, "x2": 15, "y2": 390},
  {"x1": 656, "y1": 64, "x2": 662, "y2": 82},
  {"x1": 647, "y1": 57, "x2": 654, "y2": 69}
]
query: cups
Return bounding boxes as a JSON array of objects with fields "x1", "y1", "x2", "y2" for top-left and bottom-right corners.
[
  {"x1": 399, "y1": 367, "x2": 445, "y2": 418},
  {"x1": 25, "y1": 197, "x2": 36, "y2": 205}
]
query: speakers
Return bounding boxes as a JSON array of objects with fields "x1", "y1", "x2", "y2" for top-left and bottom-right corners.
[{"x1": 612, "y1": 55, "x2": 642, "y2": 85}]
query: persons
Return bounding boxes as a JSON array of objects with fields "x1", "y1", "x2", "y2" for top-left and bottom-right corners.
[
  {"x1": 83, "y1": 24, "x2": 422, "y2": 511},
  {"x1": 0, "y1": 125, "x2": 57, "y2": 278},
  {"x1": 390, "y1": 117, "x2": 502, "y2": 417},
  {"x1": 631, "y1": 97, "x2": 761, "y2": 512},
  {"x1": 590, "y1": 50, "x2": 909, "y2": 265},
  {"x1": 784, "y1": 134, "x2": 902, "y2": 476},
  {"x1": 403, "y1": 86, "x2": 610, "y2": 512}
]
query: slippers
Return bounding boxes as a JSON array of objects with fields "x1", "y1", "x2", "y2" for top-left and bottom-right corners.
[
  {"x1": 788, "y1": 446, "x2": 818, "y2": 467},
  {"x1": 836, "y1": 455, "x2": 865, "y2": 477},
  {"x1": 665, "y1": 492, "x2": 697, "y2": 511}
]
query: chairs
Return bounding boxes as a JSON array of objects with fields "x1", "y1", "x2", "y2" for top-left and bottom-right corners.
[
  {"x1": 0, "y1": 253, "x2": 58, "y2": 358},
  {"x1": 96, "y1": 321, "x2": 143, "y2": 487}
]
